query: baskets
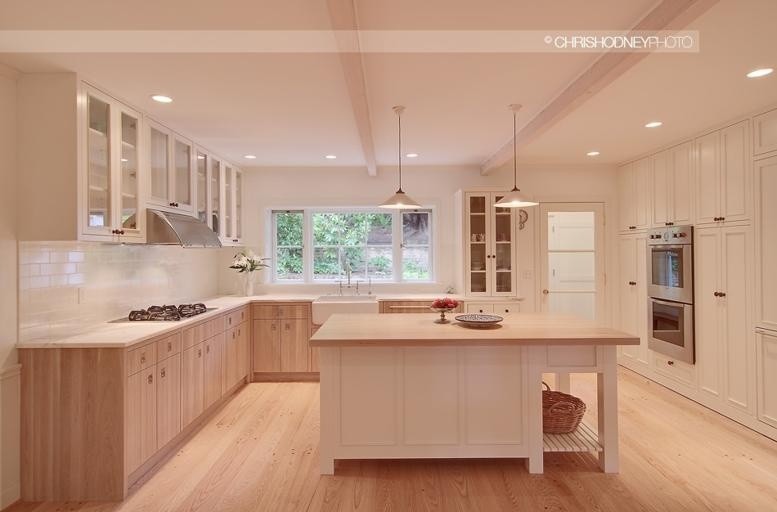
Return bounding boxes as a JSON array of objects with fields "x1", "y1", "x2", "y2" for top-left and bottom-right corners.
[{"x1": 542, "y1": 380, "x2": 587, "y2": 436}]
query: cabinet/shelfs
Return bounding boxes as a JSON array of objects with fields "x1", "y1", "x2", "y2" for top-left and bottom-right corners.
[
  {"x1": 251, "y1": 300, "x2": 308, "y2": 380},
  {"x1": 462, "y1": 298, "x2": 522, "y2": 315},
  {"x1": 123, "y1": 324, "x2": 180, "y2": 504},
  {"x1": 308, "y1": 324, "x2": 321, "y2": 382},
  {"x1": 453, "y1": 186, "x2": 518, "y2": 299},
  {"x1": 606, "y1": 104, "x2": 776, "y2": 443},
  {"x1": 224, "y1": 301, "x2": 251, "y2": 406},
  {"x1": 16, "y1": 78, "x2": 245, "y2": 247},
  {"x1": 180, "y1": 312, "x2": 224, "y2": 445}
]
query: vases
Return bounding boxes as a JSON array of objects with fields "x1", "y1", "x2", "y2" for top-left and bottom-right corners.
[{"x1": 243, "y1": 269, "x2": 255, "y2": 298}]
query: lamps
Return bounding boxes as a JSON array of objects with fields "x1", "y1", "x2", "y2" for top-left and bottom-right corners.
[
  {"x1": 375, "y1": 104, "x2": 427, "y2": 211},
  {"x1": 493, "y1": 101, "x2": 542, "y2": 208}
]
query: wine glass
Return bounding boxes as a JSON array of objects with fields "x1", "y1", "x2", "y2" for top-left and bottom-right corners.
[{"x1": 429, "y1": 307, "x2": 454, "y2": 325}]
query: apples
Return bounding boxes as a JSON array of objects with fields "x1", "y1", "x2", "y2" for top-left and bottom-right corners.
[{"x1": 433, "y1": 298, "x2": 458, "y2": 308}]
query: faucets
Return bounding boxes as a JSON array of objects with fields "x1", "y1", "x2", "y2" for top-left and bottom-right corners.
[{"x1": 347, "y1": 265, "x2": 353, "y2": 288}]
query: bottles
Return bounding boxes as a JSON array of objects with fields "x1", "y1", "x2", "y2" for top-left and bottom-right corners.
[{"x1": 473, "y1": 232, "x2": 484, "y2": 241}]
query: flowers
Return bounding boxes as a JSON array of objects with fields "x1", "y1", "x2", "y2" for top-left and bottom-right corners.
[{"x1": 227, "y1": 248, "x2": 273, "y2": 274}]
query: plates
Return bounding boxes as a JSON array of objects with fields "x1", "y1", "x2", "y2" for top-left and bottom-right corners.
[{"x1": 454, "y1": 313, "x2": 503, "y2": 329}]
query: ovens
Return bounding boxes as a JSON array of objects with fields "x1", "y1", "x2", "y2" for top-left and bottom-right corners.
[{"x1": 644, "y1": 225, "x2": 695, "y2": 366}]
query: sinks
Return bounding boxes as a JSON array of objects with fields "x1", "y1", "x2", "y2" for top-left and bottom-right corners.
[{"x1": 311, "y1": 295, "x2": 379, "y2": 325}]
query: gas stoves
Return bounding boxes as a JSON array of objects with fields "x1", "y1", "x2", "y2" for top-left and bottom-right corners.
[{"x1": 107, "y1": 303, "x2": 218, "y2": 323}]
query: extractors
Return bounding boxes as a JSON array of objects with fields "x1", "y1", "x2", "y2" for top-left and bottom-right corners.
[{"x1": 145, "y1": 208, "x2": 222, "y2": 249}]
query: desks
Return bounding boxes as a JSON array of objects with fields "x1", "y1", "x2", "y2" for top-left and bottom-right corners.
[{"x1": 306, "y1": 308, "x2": 644, "y2": 484}]
